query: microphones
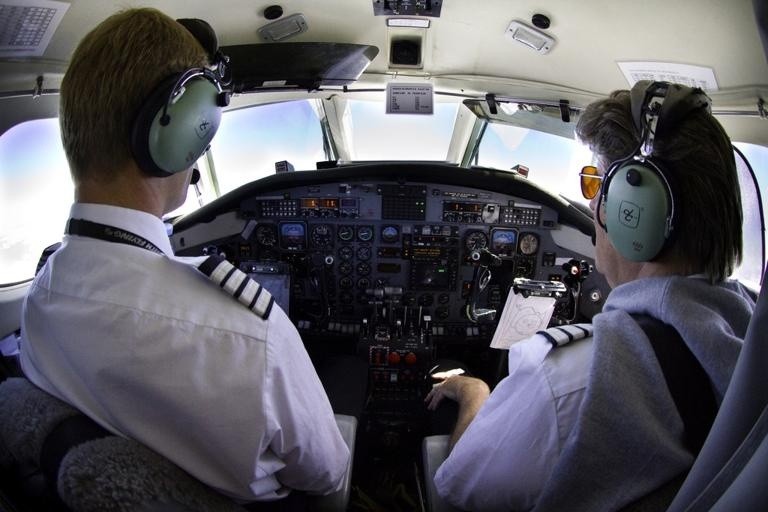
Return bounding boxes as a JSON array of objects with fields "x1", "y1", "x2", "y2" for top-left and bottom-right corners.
[
  {"x1": 190, "y1": 168, "x2": 201, "y2": 185},
  {"x1": 591, "y1": 232, "x2": 597, "y2": 246}
]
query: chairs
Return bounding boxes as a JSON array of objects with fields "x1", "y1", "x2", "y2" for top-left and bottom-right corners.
[
  {"x1": 420, "y1": 277, "x2": 767, "y2": 510},
  {"x1": 0, "y1": 374, "x2": 362, "y2": 510}
]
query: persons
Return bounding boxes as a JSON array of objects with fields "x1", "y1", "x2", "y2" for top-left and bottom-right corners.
[
  {"x1": 21, "y1": 7, "x2": 358, "y2": 507},
  {"x1": 422, "y1": 80, "x2": 761, "y2": 510}
]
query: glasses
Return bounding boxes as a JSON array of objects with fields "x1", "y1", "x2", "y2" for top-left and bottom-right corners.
[{"x1": 578, "y1": 165, "x2": 604, "y2": 200}]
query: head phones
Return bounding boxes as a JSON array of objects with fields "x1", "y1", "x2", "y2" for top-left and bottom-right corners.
[
  {"x1": 131, "y1": 51, "x2": 230, "y2": 179},
  {"x1": 594, "y1": 79, "x2": 714, "y2": 263}
]
